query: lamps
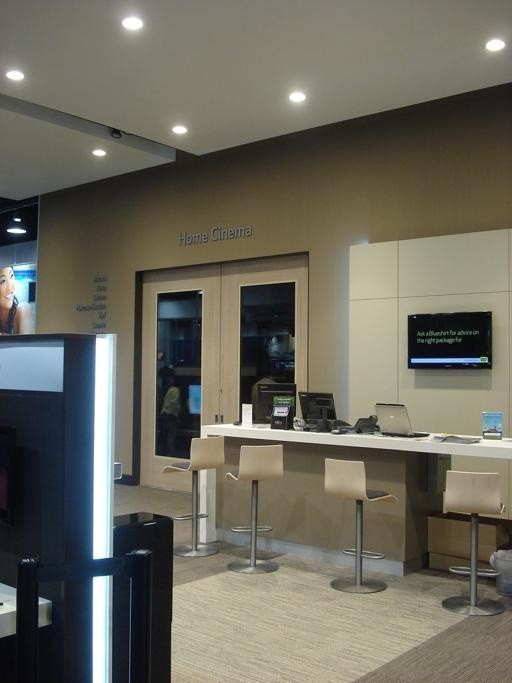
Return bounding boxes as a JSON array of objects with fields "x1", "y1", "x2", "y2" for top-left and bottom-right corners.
[{"x1": 6, "y1": 207, "x2": 28, "y2": 235}]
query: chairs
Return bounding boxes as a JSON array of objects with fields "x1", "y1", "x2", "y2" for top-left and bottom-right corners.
[
  {"x1": 161, "y1": 438, "x2": 285, "y2": 576},
  {"x1": 323, "y1": 457, "x2": 400, "y2": 595},
  {"x1": 441, "y1": 470, "x2": 508, "y2": 618}
]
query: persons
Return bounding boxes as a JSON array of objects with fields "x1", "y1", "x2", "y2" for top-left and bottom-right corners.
[
  {"x1": 249, "y1": 369, "x2": 279, "y2": 423},
  {"x1": 0, "y1": 265, "x2": 33, "y2": 336},
  {"x1": 159, "y1": 379, "x2": 183, "y2": 456}
]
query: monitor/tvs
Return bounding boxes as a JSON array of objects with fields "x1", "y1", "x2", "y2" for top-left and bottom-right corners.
[
  {"x1": 257, "y1": 384, "x2": 296, "y2": 417},
  {"x1": 408, "y1": 312, "x2": 492, "y2": 369}
]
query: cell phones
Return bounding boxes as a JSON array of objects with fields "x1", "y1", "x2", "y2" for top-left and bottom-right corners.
[{"x1": 331, "y1": 429, "x2": 347, "y2": 433}]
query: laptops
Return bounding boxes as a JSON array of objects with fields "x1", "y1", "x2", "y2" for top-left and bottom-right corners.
[
  {"x1": 298, "y1": 391, "x2": 337, "y2": 419},
  {"x1": 375, "y1": 404, "x2": 430, "y2": 437}
]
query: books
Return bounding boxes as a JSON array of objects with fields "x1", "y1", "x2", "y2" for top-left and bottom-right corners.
[{"x1": 431, "y1": 434, "x2": 481, "y2": 443}]
